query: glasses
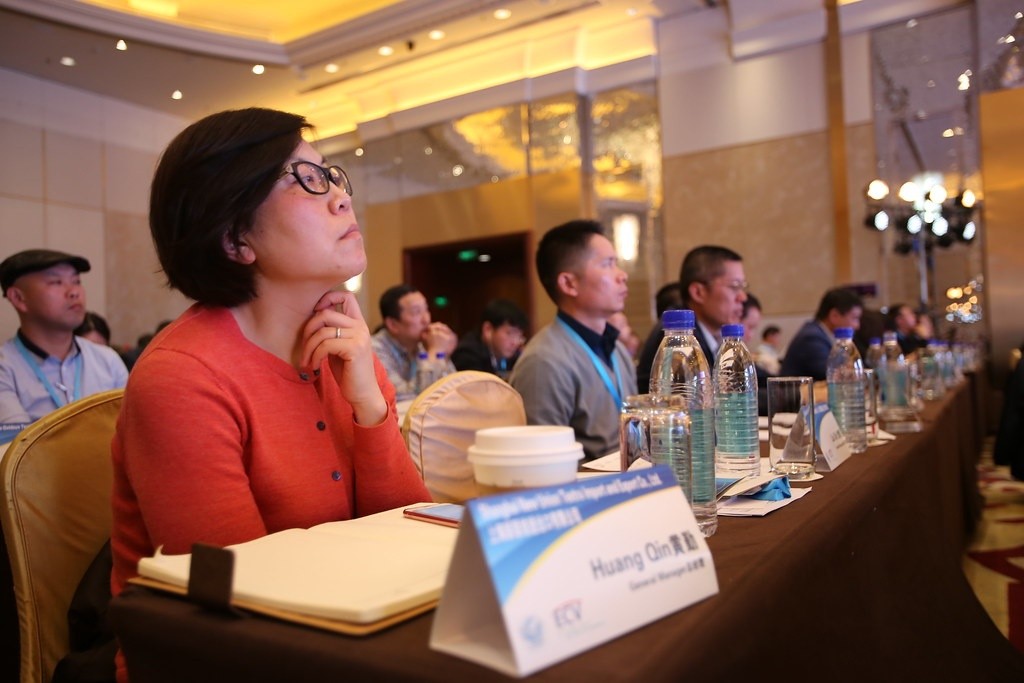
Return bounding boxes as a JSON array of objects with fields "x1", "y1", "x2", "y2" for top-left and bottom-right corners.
[{"x1": 275, "y1": 161, "x2": 352, "y2": 196}]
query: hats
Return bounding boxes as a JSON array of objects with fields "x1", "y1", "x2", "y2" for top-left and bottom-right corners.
[{"x1": 0, "y1": 249, "x2": 90, "y2": 297}]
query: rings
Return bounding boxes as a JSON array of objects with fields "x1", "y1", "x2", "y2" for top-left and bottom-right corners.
[{"x1": 336, "y1": 326, "x2": 341, "y2": 338}]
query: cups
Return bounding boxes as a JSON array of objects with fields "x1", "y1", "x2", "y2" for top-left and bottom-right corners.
[
  {"x1": 768, "y1": 377, "x2": 816, "y2": 478},
  {"x1": 904, "y1": 362, "x2": 918, "y2": 409},
  {"x1": 864, "y1": 370, "x2": 878, "y2": 439},
  {"x1": 468, "y1": 425, "x2": 585, "y2": 497},
  {"x1": 620, "y1": 395, "x2": 693, "y2": 512}
]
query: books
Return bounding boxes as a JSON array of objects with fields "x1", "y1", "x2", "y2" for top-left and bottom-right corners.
[{"x1": 130, "y1": 503, "x2": 459, "y2": 633}]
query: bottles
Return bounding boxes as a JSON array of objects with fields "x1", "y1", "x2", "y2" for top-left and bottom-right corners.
[
  {"x1": 435, "y1": 353, "x2": 447, "y2": 382},
  {"x1": 918, "y1": 340, "x2": 979, "y2": 400},
  {"x1": 649, "y1": 310, "x2": 717, "y2": 538},
  {"x1": 712, "y1": 325, "x2": 760, "y2": 479},
  {"x1": 827, "y1": 329, "x2": 867, "y2": 453},
  {"x1": 417, "y1": 354, "x2": 433, "y2": 395},
  {"x1": 864, "y1": 332, "x2": 904, "y2": 422}
]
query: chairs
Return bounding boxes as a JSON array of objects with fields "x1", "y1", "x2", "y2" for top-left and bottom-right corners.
[
  {"x1": 0, "y1": 386, "x2": 136, "y2": 681},
  {"x1": 401, "y1": 369, "x2": 528, "y2": 502}
]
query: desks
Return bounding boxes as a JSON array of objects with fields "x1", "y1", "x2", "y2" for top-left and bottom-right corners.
[{"x1": 108, "y1": 348, "x2": 989, "y2": 683}]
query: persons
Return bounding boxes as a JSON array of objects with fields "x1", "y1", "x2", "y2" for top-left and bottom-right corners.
[
  {"x1": 609, "y1": 312, "x2": 638, "y2": 356},
  {"x1": 636, "y1": 245, "x2": 856, "y2": 417},
  {"x1": 75, "y1": 312, "x2": 112, "y2": 346},
  {"x1": 507, "y1": 219, "x2": 640, "y2": 459},
  {"x1": 109, "y1": 108, "x2": 430, "y2": 682},
  {"x1": 371, "y1": 282, "x2": 457, "y2": 402},
  {"x1": 782, "y1": 287, "x2": 936, "y2": 384},
  {"x1": 451, "y1": 301, "x2": 530, "y2": 377},
  {"x1": 0, "y1": 248, "x2": 130, "y2": 458}
]
